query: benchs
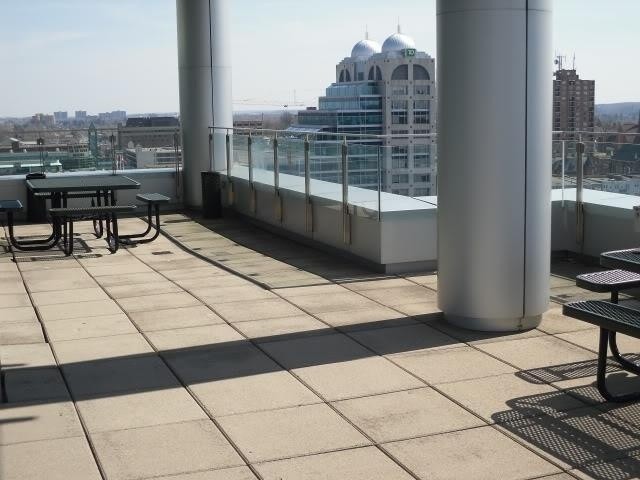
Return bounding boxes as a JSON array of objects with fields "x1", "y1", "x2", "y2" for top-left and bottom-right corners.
[
  {"x1": 561, "y1": 267, "x2": 640, "y2": 405},
  {"x1": 0, "y1": 190, "x2": 170, "y2": 257}
]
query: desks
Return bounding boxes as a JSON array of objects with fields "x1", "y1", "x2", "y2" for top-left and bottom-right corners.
[
  {"x1": 25, "y1": 174, "x2": 140, "y2": 256},
  {"x1": 601, "y1": 247, "x2": 640, "y2": 274}
]
